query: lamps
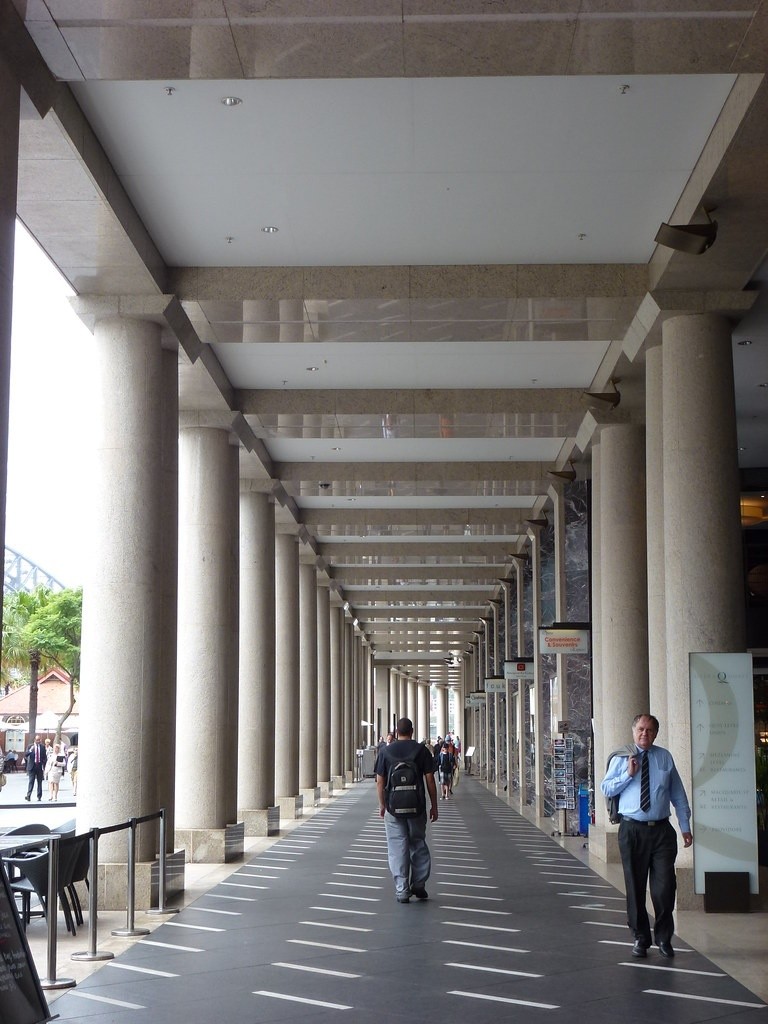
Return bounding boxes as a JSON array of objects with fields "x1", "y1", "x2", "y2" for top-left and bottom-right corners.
[
  {"x1": 654, "y1": 203, "x2": 720, "y2": 256},
  {"x1": 508, "y1": 544, "x2": 529, "y2": 566},
  {"x1": 545, "y1": 459, "x2": 578, "y2": 484},
  {"x1": 578, "y1": 378, "x2": 621, "y2": 411},
  {"x1": 523, "y1": 510, "x2": 550, "y2": 530},
  {"x1": 464, "y1": 571, "x2": 514, "y2": 655}
]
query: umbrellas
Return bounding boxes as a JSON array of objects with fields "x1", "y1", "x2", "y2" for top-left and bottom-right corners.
[{"x1": 0, "y1": 711, "x2": 79, "y2": 739}]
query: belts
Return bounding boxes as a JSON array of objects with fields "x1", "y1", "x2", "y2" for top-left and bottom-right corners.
[{"x1": 622, "y1": 816, "x2": 668, "y2": 826}]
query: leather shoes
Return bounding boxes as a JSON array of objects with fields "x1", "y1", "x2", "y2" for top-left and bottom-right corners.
[
  {"x1": 658, "y1": 938, "x2": 675, "y2": 959},
  {"x1": 631, "y1": 938, "x2": 648, "y2": 956}
]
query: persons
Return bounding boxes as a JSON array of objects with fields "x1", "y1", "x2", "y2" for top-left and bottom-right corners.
[
  {"x1": 375, "y1": 734, "x2": 395, "y2": 782},
  {"x1": 600, "y1": 714, "x2": 692, "y2": 959},
  {"x1": 25, "y1": 736, "x2": 78, "y2": 802},
  {"x1": 421, "y1": 730, "x2": 461, "y2": 800},
  {"x1": 373, "y1": 717, "x2": 438, "y2": 903},
  {"x1": 0, "y1": 746, "x2": 18, "y2": 792}
]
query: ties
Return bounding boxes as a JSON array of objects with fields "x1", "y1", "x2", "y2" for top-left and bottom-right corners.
[
  {"x1": 36, "y1": 744, "x2": 39, "y2": 768},
  {"x1": 640, "y1": 750, "x2": 651, "y2": 813}
]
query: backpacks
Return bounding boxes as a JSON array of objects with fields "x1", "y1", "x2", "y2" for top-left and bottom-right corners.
[{"x1": 381, "y1": 742, "x2": 422, "y2": 817}]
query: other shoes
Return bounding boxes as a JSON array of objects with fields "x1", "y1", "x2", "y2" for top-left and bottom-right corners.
[
  {"x1": 411, "y1": 886, "x2": 428, "y2": 898},
  {"x1": 37, "y1": 796, "x2": 41, "y2": 801},
  {"x1": 25, "y1": 796, "x2": 30, "y2": 801},
  {"x1": 397, "y1": 895, "x2": 409, "y2": 903},
  {"x1": 450, "y1": 789, "x2": 453, "y2": 794},
  {"x1": 444, "y1": 796, "x2": 449, "y2": 800},
  {"x1": 440, "y1": 796, "x2": 445, "y2": 800}
]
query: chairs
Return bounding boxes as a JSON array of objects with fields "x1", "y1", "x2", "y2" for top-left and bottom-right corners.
[{"x1": 0, "y1": 817, "x2": 100, "y2": 938}]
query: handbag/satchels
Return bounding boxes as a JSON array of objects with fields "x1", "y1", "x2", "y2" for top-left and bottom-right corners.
[{"x1": 54, "y1": 756, "x2": 63, "y2": 766}]
